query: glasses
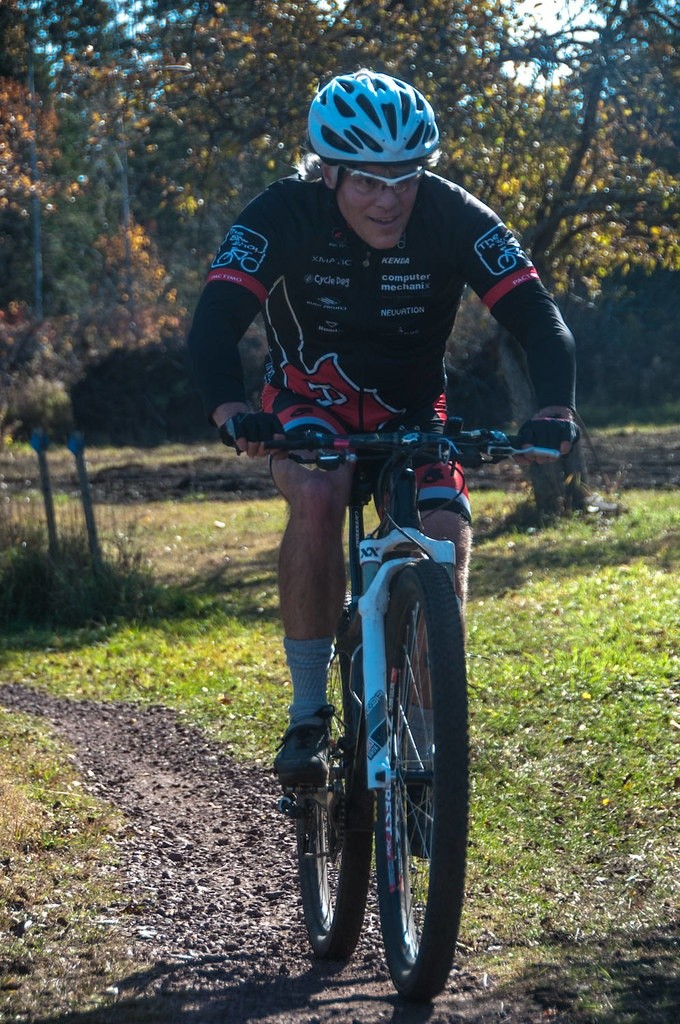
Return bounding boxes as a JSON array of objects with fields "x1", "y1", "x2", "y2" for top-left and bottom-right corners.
[{"x1": 339, "y1": 162, "x2": 426, "y2": 195}]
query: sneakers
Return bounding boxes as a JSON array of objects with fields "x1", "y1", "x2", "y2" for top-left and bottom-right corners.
[
  {"x1": 274, "y1": 705, "x2": 335, "y2": 787},
  {"x1": 403, "y1": 767, "x2": 431, "y2": 859}
]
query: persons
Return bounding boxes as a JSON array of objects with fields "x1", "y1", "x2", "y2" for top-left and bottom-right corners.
[{"x1": 186, "y1": 68, "x2": 576, "y2": 858}]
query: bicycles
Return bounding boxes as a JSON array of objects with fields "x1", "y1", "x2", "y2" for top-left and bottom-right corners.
[{"x1": 254, "y1": 417, "x2": 520, "y2": 1001}]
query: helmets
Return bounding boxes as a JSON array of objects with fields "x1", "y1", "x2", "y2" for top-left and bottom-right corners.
[{"x1": 308, "y1": 69, "x2": 439, "y2": 165}]
